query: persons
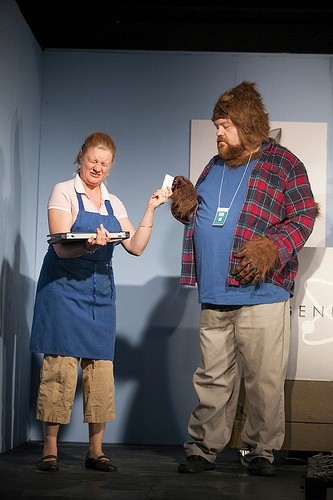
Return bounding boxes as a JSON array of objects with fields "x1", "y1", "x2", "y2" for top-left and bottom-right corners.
[
  {"x1": 29, "y1": 131, "x2": 173, "y2": 473},
  {"x1": 171, "y1": 79, "x2": 319, "y2": 477}
]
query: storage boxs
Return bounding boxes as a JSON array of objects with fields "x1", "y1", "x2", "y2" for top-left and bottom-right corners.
[{"x1": 227, "y1": 378, "x2": 333, "y2": 452}]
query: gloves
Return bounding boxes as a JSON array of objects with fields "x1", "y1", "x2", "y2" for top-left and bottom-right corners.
[
  {"x1": 232, "y1": 235, "x2": 279, "y2": 286},
  {"x1": 170, "y1": 176, "x2": 198, "y2": 221}
]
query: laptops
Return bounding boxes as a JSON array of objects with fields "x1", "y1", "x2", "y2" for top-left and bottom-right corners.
[{"x1": 47, "y1": 232, "x2": 130, "y2": 244}]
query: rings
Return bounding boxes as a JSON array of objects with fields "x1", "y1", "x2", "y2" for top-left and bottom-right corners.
[{"x1": 163, "y1": 195, "x2": 166, "y2": 198}]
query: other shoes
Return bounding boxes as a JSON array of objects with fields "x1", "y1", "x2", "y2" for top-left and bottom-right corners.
[
  {"x1": 247, "y1": 457, "x2": 276, "y2": 477},
  {"x1": 85, "y1": 456, "x2": 117, "y2": 472},
  {"x1": 177, "y1": 455, "x2": 215, "y2": 473},
  {"x1": 38, "y1": 455, "x2": 57, "y2": 471}
]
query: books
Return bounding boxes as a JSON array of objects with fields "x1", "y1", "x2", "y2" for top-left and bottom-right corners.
[{"x1": 45, "y1": 232, "x2": 131, "y2": 243}]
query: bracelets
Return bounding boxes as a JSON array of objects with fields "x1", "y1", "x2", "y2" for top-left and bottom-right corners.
[{"x1": 139, "y1": 223, "x2": 153, "y2": 229}]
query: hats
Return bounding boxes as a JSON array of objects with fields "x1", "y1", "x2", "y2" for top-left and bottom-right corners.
[{"x1": 211, "y1": 80, "x2": 270, "y2": 153}]
